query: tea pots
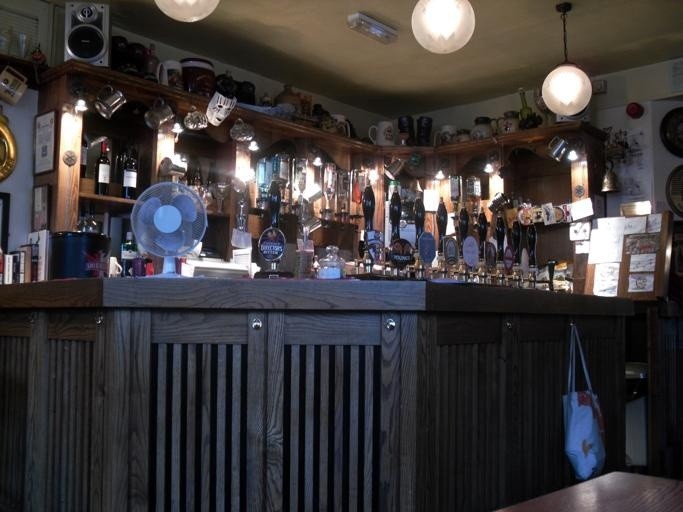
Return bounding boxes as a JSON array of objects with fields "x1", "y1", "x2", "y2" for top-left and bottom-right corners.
[
  {"x1": 470, "y1": 116, "x2": 498, "y2": 141},
  {"x1": 433, "y1": 124, "x2": 458, "y2": 147},
  {"x1": 497, "y1": 111, "x2": 521, "y2": 134}
]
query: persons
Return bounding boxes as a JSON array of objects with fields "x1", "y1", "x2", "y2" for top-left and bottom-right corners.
[
  {"x1": 264, "y1": 229, "x2": 281, "y2": 245},
  {"x1": 627, "y1": 234, "x2": 656, "y2": 253}
]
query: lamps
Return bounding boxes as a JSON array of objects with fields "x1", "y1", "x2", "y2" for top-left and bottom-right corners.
[
  {"x1": 541, "y1": 1, "x2": 592, "y2": 116},
  {"x1": 347, "y1": 12, "x2": 401, "y2": 46},
  {"x1": 153, "y1": 0, "x2": 221, "y2": 23},
  {"x1": 411, "y1": 0, "x2": 476, "y2": 55}
]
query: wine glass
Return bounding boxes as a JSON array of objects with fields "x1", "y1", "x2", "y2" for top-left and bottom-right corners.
[{"x1": 213, "y1": 182, "x2": 228, "y2": 213}]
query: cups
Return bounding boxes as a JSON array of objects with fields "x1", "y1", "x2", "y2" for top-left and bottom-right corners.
[
  {"x1": 157, "y1": 60, "x2": 183, "y2": 89},
  {"x1": 183, "y1": 106, "x2": 209, "y2": 131},
  {"x1": 331, "y1": 114, "x2": 351, "y2": 138},
  {"x1": 0, "y1": 66, "x2": 28, "y2": 106},
  {"x1": 18, "y1": 32, "x2": 29, "y2": 58},
  {"x1": 95, "y1": 85, "x2": 128, "y2": 120},
  {"x1": 144, "y1": 98, "x2": 175, "y2": 131},
  {"x1": 108, "y1": 255, "x2": 123, "y2": 278},
  {"x1": 548, "y1": 136, "x2": 570, "y2": 162},
  {"x1": 369, "y1": 120, "x2": 395, "y2": 147},
  {"x1": 384, "y1": 158, "x2": 405, "y2": 181},
  {"x1": 229, "y1": 119, "x2": 255, "y2": 142}
]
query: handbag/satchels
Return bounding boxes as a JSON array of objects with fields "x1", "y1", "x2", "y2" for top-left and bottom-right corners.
[{"x1": 562, "y1": 325, "x2": 606, "y2": 481}]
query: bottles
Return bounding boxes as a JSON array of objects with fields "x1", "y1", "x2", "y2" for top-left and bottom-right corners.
[
  {"x1": 186, "y1": 164, "x2": 194, "y2": 186},
  {"x1": 466, "y1": 177, "x2": 482, "y2": 215},
  {"x1": 450, "y1": 175, "x2": 461, "y2": 213},
  {"x1": 145, "y1": 43, "x2": 160, "y2": 80},
  {"x1": 257, "y1": 152, "x2": 365, "y2": 216},
  {"x1": 78, "y1": 206, "x2": 89, "y2": 232},
  {"x1": 260, "y1": 92, "x2": 273, "y2": 107},
  {"x1": 88, "y1": 203, "x2": 100, "y2": 233},
  {"x1": 207, "y1": 163, "x2": 216, "y2": 186},
  {"x1": 95, "y1": 142, "x2": 110, "y2": 196},
  {"x1": 80, "y1": 125, "x2": 89, "y2": 178},
  {"x1": 124, "y1": 149, "x2": 137, "y2": 199},
  {"x1": 120, "y1": 231, "x2": 140, "y2": 277},
  {"x1": 518, "y1": 88, "x2": 533, "y2": 118},
  {"x1": 193, "y1": 162, "x2": 202, "y2": 185}
]
